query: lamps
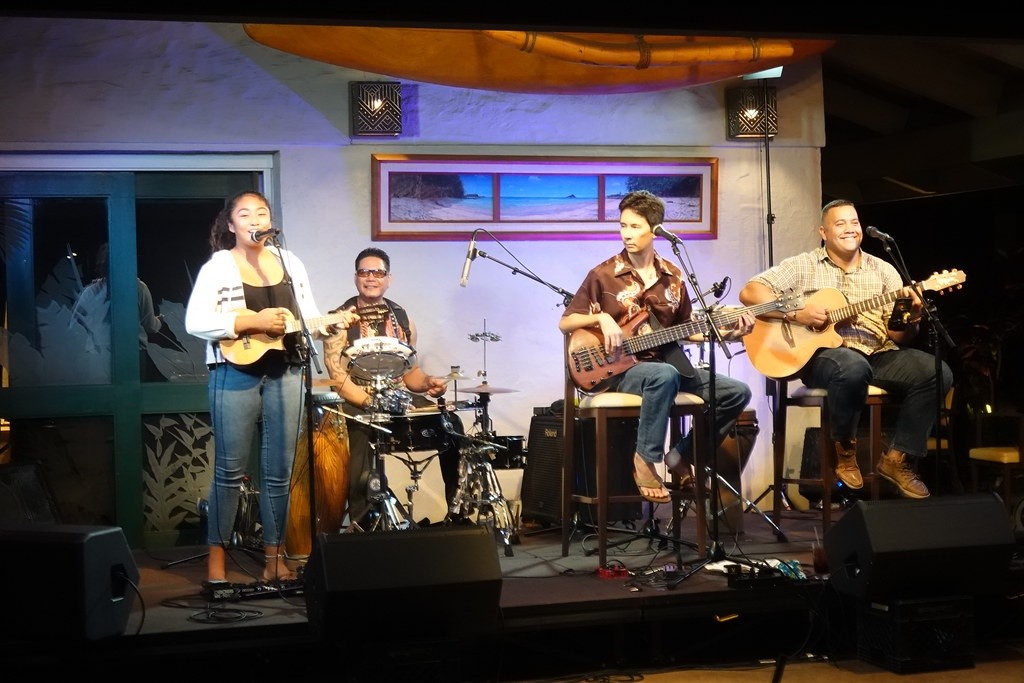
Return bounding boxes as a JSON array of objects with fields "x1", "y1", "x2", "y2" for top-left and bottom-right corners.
[
  {"x1": 724, "y1": 84, "x2": 778, "y2": 140},
  {"x1": 350, "y1": 80, "x2": 402, "y2": 137}
]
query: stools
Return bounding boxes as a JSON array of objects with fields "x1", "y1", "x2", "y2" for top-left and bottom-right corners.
[{"x1": 969, "y1": 445, "x2": 1024, "y2": 514}]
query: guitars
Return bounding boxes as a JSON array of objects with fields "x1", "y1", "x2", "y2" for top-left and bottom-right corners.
[
  {"x1": 217, "y1": 302, "x2": 392, "y2": 370},
  {"x1": 565, "y1": 286, "x2": 807, "y2": 397},
  {"x1": 742, "y1": 267, "x2": 968, "y2": 384}
]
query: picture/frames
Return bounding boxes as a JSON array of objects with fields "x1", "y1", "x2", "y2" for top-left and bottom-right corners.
[{"x1": 370, "y1": 154, "x2": 718, "y2": 240}]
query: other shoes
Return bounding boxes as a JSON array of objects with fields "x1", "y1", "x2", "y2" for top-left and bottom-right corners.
[{"x1": 447, "y1": 511, "x2": 476, "y2": 528}]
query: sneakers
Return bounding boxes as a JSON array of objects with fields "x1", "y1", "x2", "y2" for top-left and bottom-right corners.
[
  {"x1": 876, "y1": 449, "x2": 931, "y2": 499},
  {"x1": 834, "y1": 440, "x2": 864, "y2": 489}
]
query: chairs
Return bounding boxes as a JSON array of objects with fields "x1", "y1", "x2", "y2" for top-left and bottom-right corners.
[
  {"x1": 561, "y1": 331, "x2": 706, "y2": 571},
  {"x1": 772, "y1": 380, "x2": 891, "y2": 548}
]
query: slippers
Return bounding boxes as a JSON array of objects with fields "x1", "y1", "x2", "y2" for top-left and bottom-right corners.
[
  {"x1": 631, "y1": 466, "x2": 671, "y2": 504},
  {"x1": 261, "y1": 573, "x2": 298, "y2": 583},
  {"x1": 663, "y1": 475, "x2": 711, "y2": 494}
]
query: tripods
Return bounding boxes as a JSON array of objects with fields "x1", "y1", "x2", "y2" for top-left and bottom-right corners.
[
  {"x1": 312, "y1": 248, "x2": 801, "y2": 590},
  {"x1": 161, "y1": 494, "x2": 265, "y2": 569}
]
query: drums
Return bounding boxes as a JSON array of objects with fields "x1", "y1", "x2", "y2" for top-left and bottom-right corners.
[
  {"x1": 285, "y1": 390, "x2": 354, "y2": 564},
  {"x1": 368, "y1": 410, "x2": 450, "y2": 453},
  {"x1": 457, "y1": 434, "x2": 525, "y2": 470},
  {"x1": 707, "y1": 408, "x2": 760, "y2": 535},
  {"x1": 337, "y1": 303, "x2": 417, "y2": 382}
]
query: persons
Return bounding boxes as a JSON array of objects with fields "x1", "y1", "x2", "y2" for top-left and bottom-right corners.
[
  {"x1": 560, "y1": 189, "x2": 757, "y2": 503},
  {"x1": 71, "y1": 246, "x2": 162, "y2": 381},
  {"x1": 322, "y1": 249, "x2": 475, "y2": 534},
  {"x1": 739, "y1": 198, "x2": 954, "y2": 500},
  {"x1": 182, "y1": 192, "x2": 360, "y2": 587}
]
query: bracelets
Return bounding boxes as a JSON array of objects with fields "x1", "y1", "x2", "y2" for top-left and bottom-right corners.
[{"x1": 793, "y1": 310, "x2": 796, "y2": 320}]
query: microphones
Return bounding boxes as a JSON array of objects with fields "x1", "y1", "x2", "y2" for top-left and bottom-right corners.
[
  {"x1": 866, "y1": 226, "x2": 894, "y2": 241},
  {"x1": 461, "y1": 230, "x2": 477, "y2": 287},
  {"x1": 652, "y1": 224, "x2": 683, "y2": 244},
  {"x1": 714, "y1": 277, "x2": 728, "y2": 298},
  {"x1": 251, "y1": 228, "x2": 279, "y2": 243}
]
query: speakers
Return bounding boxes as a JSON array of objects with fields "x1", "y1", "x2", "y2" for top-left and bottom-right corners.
[
  {"x1": 519, "y1": 416, "x2": 640, "y2": 522},
  {"x1": 302, "y1": 524, "x2": 503, "y2": 622},
  {"x1": 0, "y1": 520, "x2": 142, "y2": 640},
  {"x1": 823, "y1": 491, "x2": 1018, "y2": 597},
  {"x1": 799, "y1": 427, "x2": 921, "y2": 503}
]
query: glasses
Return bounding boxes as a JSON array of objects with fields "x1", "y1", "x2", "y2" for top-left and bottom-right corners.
[{"x1": 357, "y1": 269, "x2": 388, "y2": 278}]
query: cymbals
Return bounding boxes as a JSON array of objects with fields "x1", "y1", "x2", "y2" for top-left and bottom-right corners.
[
  {"x1": 428, "y1": 373, "x2": 474, "y2": 381},
  {"x1": 450, "y1": 386, "x2": 520, "y2": 394}
]
